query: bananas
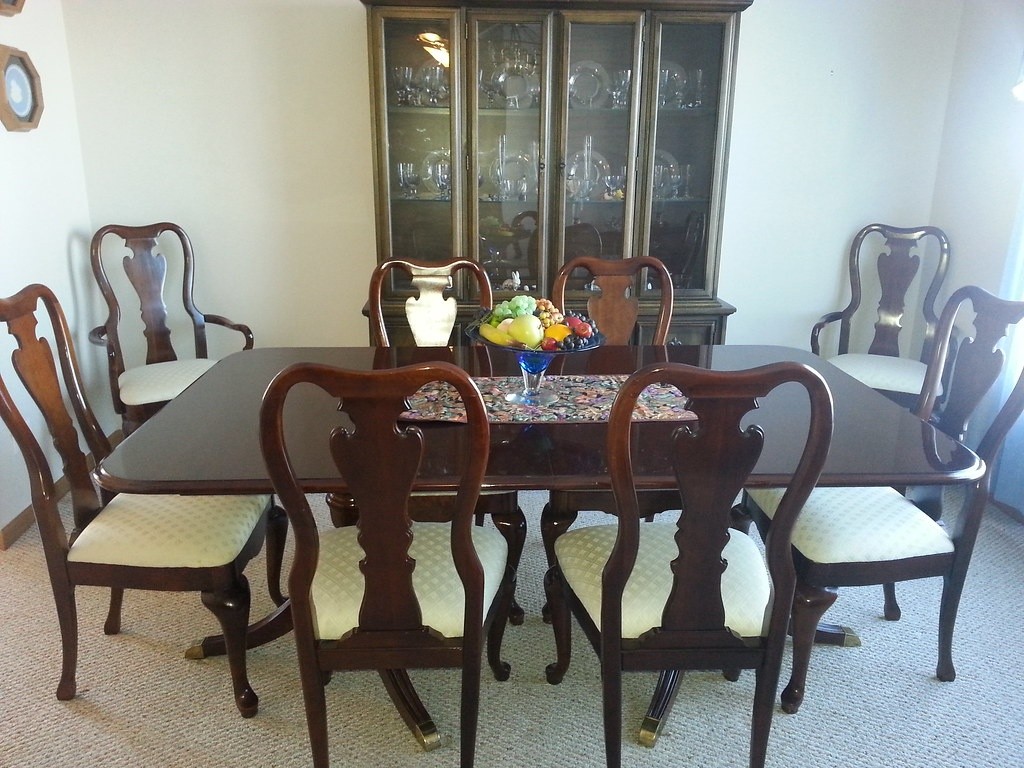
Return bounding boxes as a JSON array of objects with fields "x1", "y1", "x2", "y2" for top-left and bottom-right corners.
[{"x1": 479, "y1": 324, "x2": 527, "y2": 349}]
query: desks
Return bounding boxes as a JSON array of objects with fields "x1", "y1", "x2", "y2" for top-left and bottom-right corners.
[{"x1": 93, "y1": 344, "x2": 987, "y2": 660}]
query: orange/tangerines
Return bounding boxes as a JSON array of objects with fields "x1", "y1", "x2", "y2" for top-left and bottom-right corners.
[{"x1": 545, "y1": 324, "x2": 573, "y2": 342}]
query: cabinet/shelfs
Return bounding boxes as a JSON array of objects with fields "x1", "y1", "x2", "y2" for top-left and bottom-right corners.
[{"x1": 361, "y1": 0, "x2": 754, "y2": 348}]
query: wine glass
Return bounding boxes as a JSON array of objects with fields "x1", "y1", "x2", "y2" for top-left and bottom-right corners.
[
  {"x1": 397, "y1": 157, "x2": 694, "y2": 201},
  {"x1": 390, "y1": 66, "x2": 710, "y2": 109}
]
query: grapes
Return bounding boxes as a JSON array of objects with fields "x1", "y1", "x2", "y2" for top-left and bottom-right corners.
[{"x1": 473, "y1": 295, "x2": 599, "y2": 351}]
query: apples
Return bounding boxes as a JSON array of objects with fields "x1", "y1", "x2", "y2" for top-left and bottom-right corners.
[
  {"x1": 540, "y1": 337, "x2": 557, "y2": 351},
  {"x1": 551, "y1": 312, "x2": 564, "y2": 324},
  {"x1": 563, "y1": 317, "x2": 583, "y2": 330},
  {"x1": 575, "y1": 323, "x2": 593, "y2": 339},
  {"x1": 507, "y1": 314, "x2": 544, "y2": 348}
]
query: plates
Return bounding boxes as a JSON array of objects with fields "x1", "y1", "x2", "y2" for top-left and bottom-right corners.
[
  {"x1": 419, "y1": 151, "x2": 452, "y2": 194},
  {"x1": 415, "y1": 59, "x2": 452, "y2": 108},
  {"x1": 570, "y1": 61, "x2": 609, "y2": 110},
  {"x1": 659, "y1": 60, "x2": 690, "y2": 109},
  {"x1": 490, "y1": 153, "x2": 532, "y2": 195},
  {"x1": 492, "y1": 60, "x2": 539, "y2": 110},
  {"x1": 654, "y1": 149, "x2": 679, "y2": 197},
  {"x1": 566, "y1": 150, "x2": 610, "y2": 199}
]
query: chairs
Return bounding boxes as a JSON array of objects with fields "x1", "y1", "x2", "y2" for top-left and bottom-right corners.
[
  {"x1": 88, "y1": 222, "x2": 254, "y2": 438},
  {"x1": 368, "y1": 257, "x2": 492, "y2": 347},
  {"x1": 810, "y1": 223, "x2": 960, "y2": 424},
  {"x1": 732, "y1": 286, "x2": 1024, "y2": 714},
  {"x1": 0, "y1": 283, "x2": 291, "y2": 718},
  {"x1": 553, "y1": 255, "x2": 674, "y2": 345},
  {"x1": 258, "y1": 362, "x2": 511, "y2": 768},
  {"x1": 543, "y1": 362, "x2": 835, "y2": 768}
]
